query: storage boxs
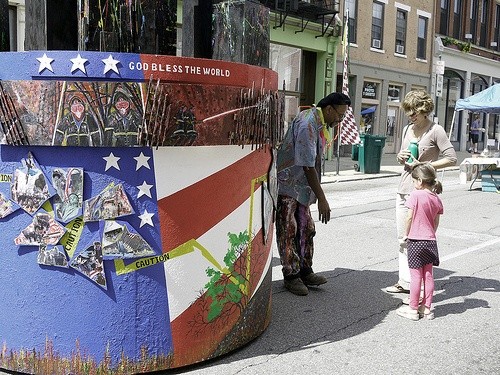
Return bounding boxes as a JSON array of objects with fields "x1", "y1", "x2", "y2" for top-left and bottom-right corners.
[{"x1": 481, "y1": 170, "x2": 500, "y2": 192}]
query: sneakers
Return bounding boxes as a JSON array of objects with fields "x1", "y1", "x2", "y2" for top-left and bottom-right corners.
[
  {"x1": 284, "y1": 278, "x2": 309, "y2": 296},
  {"x1": 302, "y1": 273, "x2": 327, "y2": 286}
]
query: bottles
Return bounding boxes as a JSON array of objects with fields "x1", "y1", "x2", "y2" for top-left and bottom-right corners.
[{"x1": 407, "y1": 140, "x2": 418, "y2": 163}]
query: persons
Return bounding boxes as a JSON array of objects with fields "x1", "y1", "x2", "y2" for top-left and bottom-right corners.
[
  {"x1": 395, "y1": 163, "x2": 444, "y2": 321},
  {"x1": 386, "y1": 88, "x2": 458, "y2": 305},
  {"x1": 467, "y1": 117, "x2": 483, "y2": 153},
  {"x1": 276, "y1": 92, "x2": 352, "y2": 297}
]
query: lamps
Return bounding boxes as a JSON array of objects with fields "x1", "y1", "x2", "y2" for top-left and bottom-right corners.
[
  {"x1": 465, "y1": 33, "x2": 472, "y2": 42},
  {"x1": 490, "y1": 41, "x2": 498, "y2": 59}
]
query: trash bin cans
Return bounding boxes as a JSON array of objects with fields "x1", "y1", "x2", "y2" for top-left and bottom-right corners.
[{"x1": 351, "y1": 132, "x2": 387, "y2": 175}]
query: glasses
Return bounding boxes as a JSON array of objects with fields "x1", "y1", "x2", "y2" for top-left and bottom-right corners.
[{"x1": 332, "y1": 105, "x2": 345, "y2": 119}]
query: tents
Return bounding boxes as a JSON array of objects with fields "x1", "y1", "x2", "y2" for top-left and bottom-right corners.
[{"x1": 449, "y1": 83, "x2": 500, "y2": 142}]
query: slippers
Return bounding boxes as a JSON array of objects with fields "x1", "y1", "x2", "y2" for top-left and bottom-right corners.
[
  {"x1": 402, "y1": 297, "x2": 422, "y2": 306},
  {"x1": 386, "y1": 284, "x2": 410, "y2": 294},
  {"x1": 396, "y1": 306, "x2": 419, "y2": 321},
  {"x1": 418, "y1": 306, "x2": 434, "y2": 319}
]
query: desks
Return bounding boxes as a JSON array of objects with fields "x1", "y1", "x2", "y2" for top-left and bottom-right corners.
[{"x1": 458, "y1": 157, "x2": 500, "y2": 192}]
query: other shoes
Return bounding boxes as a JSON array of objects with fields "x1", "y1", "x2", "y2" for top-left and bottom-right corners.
[
  {"x1": 469, "y1": 147, "x2": 473, "y2": 150},
  {"x1": 475, "y1": 148, "x2": 477, "y2": 153}
]
